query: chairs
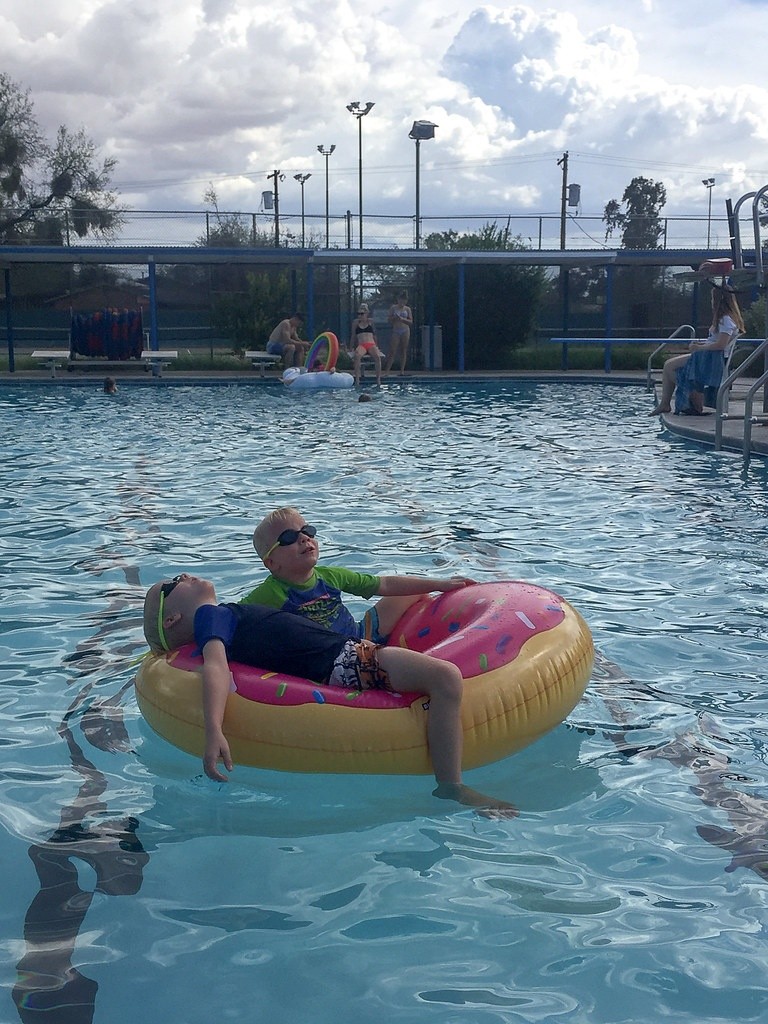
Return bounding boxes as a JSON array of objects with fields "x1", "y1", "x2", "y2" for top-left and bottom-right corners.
[{"x1": 677, "y1": 331, "x2": 745, "y2": 415}]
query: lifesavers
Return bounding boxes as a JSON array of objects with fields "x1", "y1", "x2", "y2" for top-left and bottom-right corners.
[
  {"x1": 287, "y1": 370, "x2": 355, "y2": 388},
  {"x1": 135, "y1": 580, "x2": 595, "y2": 777}
]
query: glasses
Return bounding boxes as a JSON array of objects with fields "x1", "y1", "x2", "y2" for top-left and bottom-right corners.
[
  {"x1": 357, "y1": 313, "x2": 365, "y2": 315},
  {"x1": 158, "y1": 575, "x2": 181, "y2": 651},
  {"x1": 262, "y1": 525, "x2": 316, "y2": 560}
]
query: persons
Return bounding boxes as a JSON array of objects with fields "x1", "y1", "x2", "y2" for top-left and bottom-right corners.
[
  {"x1": 236, "y1": 508, "x2": 477, "y2": 646},
  {"x1": 307, "y1": 359, "x2": 335, "y2": 373},
  {"x1": 383, "y1": 290, "x2": 413, "y2": 376},
  {"x1": 648, "y1": 285, "x2": 744, "y2": 417},
  {"x1": 349, "y1": 303, "x2": 381, "y2": 385},
  {"x1": 103, "y1": 377, "x2": 118, "y2": 393},
  {"x1": 144, "y1": 573, "x2": 520, "y2": 820},
  {"x1": 358, "y1": 393, "x2": 372, "y2": 403},
  {"x1": 266, "y1": 312, "x2": 311, "y2": 370}
]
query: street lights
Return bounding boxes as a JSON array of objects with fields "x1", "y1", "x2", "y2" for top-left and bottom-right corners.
[
  {"x1": 294, "y1": 172, "x2": 312, "y2": 249},
  {"x1": 409, "y1": 120, "x2": 441, "y2": 249},
  {"x1": 316, "y1": 143, "x2": 336, "y2": 248},
  {"x1": 701, "y1": 177, "x2": 716, "y2": 249},
  {"x1": 346, "y1": 100, "x2": 376, "y2": 300}
]
